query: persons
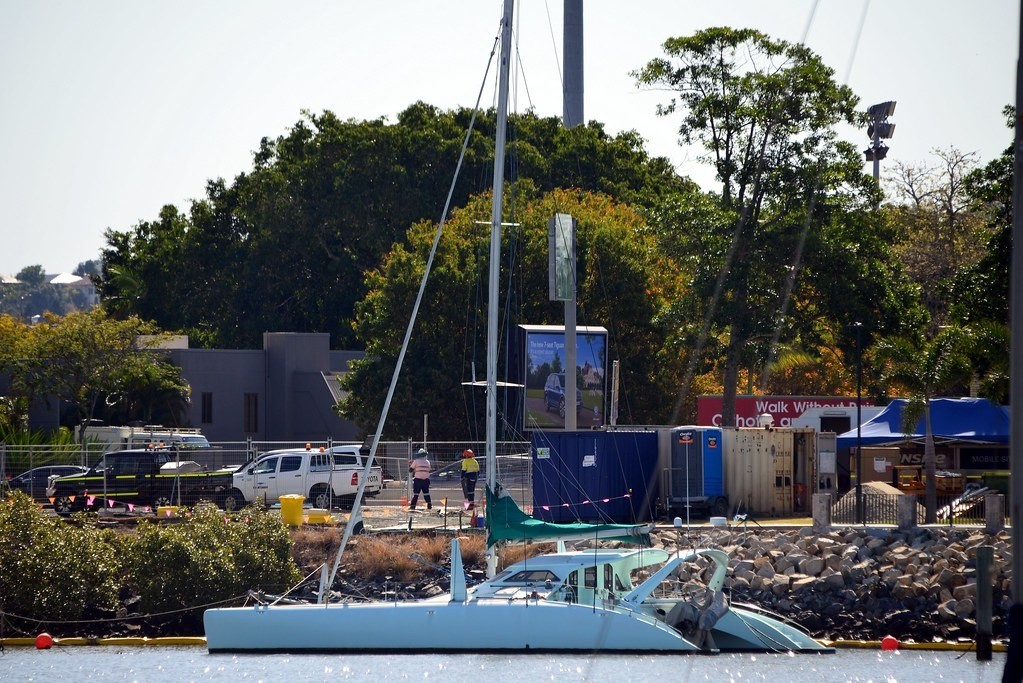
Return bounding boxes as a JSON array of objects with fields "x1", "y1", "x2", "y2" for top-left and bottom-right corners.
[
  {"x1": 409, "y1": 448, "x2": 432, "y2": 511},
  {"x1": 459, "y1": 448, "x2": 480, "y2": 506}
]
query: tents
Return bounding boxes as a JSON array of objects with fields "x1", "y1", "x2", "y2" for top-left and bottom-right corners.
[{"x1": 836, "y1": 398, "x2": 1011, "y2": 453}]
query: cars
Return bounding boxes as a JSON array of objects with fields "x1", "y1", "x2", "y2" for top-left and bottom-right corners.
[{"x1": 0, "y1": 464, "x2": 93, "y2": 509}]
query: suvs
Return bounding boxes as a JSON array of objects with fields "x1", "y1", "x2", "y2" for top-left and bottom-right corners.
[
  {"x1": 545, "y1": 372, "x2": 584, "y2": 419},
  {"x1": 228, "y1": 446, "x2": 381, "y2": 474}
]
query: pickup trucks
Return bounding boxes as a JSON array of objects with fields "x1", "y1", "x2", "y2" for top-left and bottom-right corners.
[
  {"x1": 44, "y1": 447, "x2": 235, "y2": 517},
  {"x1": 217, "y1": 443, "x2": 384, "y2": 507}
]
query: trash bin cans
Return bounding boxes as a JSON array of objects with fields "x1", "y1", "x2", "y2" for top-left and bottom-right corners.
[{"x1": 279, "y1": 494, "x2": 305, "y2": 527}]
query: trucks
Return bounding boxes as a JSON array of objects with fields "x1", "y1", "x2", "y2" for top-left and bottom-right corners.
[{"x1": 75, "y1": 424, "x2": 210, "y2": 451}]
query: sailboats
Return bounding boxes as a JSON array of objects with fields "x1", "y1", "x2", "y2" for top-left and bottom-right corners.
[{"x1": 199, "y1": 0, "x2": 840, "y2": 657}]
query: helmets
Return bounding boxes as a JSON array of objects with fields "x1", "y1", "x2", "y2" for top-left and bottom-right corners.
[
  {"x1": 417, "y1": 448, "x2": 427, "y2": 456},
  {"x1": 464, "y1": 449, "x2": 475, "y2": 457}
]
